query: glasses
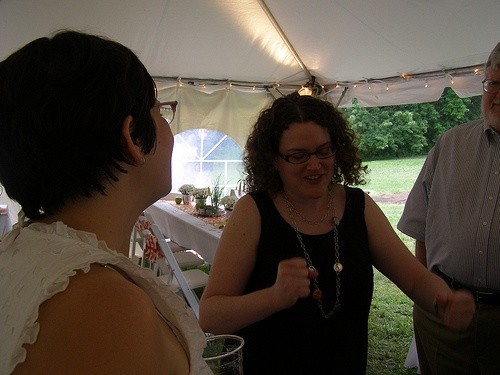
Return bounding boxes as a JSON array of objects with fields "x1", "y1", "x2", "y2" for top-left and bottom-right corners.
[
  {"x1": 278, "y1": 144, "x2": 338, "y2": 164},
  {"x1": 482, "y1": 78, "x2": 500, "y2": 94},
  {"x1": 160, "y1": 100, "x2": 178, "y2": 125}
]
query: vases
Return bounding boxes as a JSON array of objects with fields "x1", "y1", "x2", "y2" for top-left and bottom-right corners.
[
  {"x1": 183, "y1": 195, "x2": 192, "y2": 205},
  {"x1": 196, "y1": 199, "x2": 205, "y2": 210}
]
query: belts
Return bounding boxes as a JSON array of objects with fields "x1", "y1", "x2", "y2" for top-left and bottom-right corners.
[{"x1": 431, "y1": 264, "x2": 500, "y2": 305}]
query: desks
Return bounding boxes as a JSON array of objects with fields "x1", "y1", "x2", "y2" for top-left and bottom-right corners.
[{"x1": 144, "y1": 200, "x2": 233, "y2": 263}]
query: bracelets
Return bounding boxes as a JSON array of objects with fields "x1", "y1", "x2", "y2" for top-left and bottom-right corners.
[{"x1": 433, "y1": 298, "x2": 440, "y2": 319}]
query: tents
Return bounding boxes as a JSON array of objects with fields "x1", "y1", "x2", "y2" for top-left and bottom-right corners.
[{"x1": 0, "y1": 0, "x2": 500, "y2": 266}]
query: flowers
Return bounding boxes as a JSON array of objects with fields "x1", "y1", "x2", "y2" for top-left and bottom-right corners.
[
  {"x1": 193, "y1": 187, "x2": 212, "y2": 200},
  {"x1": 179, "y1": 184, "x2": 196, "y2": 196}
]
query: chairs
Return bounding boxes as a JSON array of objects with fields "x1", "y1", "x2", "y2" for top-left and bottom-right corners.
[{"x1": 129, "y1": 213, "x2": 210, "y2": 295}]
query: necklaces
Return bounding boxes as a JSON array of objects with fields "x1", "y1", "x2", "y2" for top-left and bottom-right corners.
[{"x1": 286, "y1": 190, "x2": 343, "y2": 319}]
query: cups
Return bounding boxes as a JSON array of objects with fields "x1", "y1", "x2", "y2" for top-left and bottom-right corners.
[
  {"x1": 175, "y1": 198, "x2": 182, "y2": 204},
  {"x1": 202, "y1": 335, "x2": 244, "y2": 375}
]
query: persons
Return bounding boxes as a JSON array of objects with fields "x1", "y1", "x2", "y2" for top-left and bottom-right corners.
[
  {"x1": 0, "y1": 30, "x2": 214, "y2": 375},
  {"x1": 199, "y1": 91, "x2": 474, "y2": 374},
  {"x1": 398, "y1": 43, "x2": 500, "y2": 375}
]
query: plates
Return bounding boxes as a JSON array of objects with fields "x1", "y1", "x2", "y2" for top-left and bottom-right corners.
[{"x1": 196, "y1": 210, "x2": 227, "y2": 217}]
